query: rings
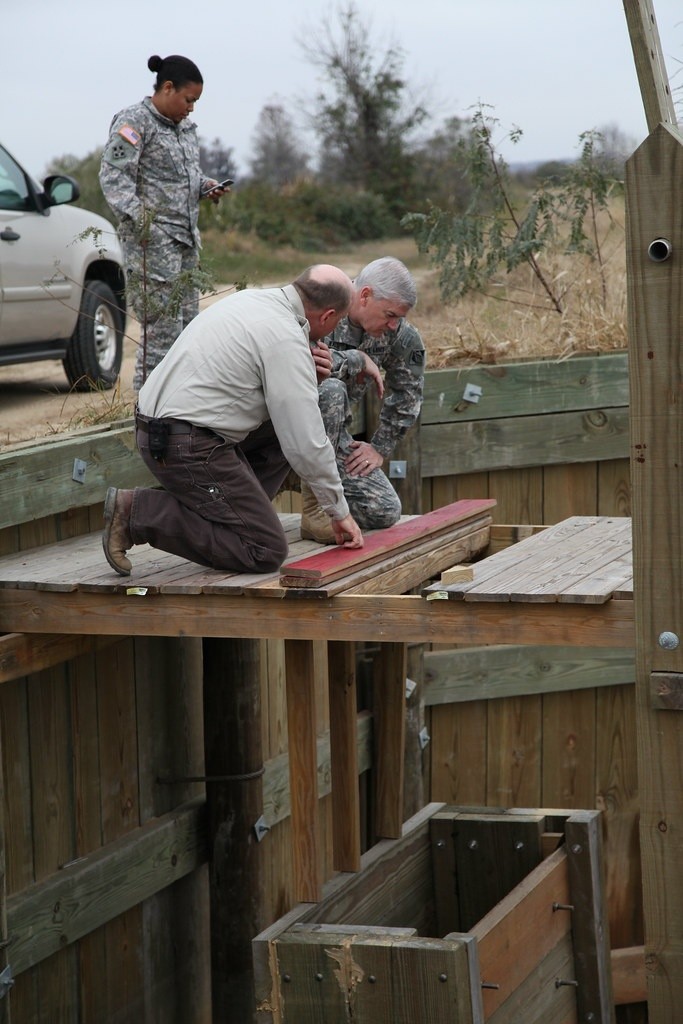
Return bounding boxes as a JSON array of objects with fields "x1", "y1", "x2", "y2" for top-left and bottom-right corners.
[{"x1": 365, "y1": 459, "x2": 369, "y2": 466}]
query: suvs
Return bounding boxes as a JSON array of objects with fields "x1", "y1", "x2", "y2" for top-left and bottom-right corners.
[{"x1": 0, "y1": 143, "x2": 129, "y2": 393}]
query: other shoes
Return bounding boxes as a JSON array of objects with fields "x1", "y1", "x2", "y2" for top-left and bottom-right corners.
[
  {"x1": 101, "y1": 487, "x2": 132, "y2": 576},
  {"x1": 300, "y1": 491, "x2": 353, "y2": 544}
]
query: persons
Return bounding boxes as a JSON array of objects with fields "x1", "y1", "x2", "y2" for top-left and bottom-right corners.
[
  {"x1": 279, "y1": 257, "x2": 426, "y2": 532},
  {"x1": 98, "y1": 54, "x2": 231, "y2": 397},
  {"x1": 101, "y1": 264, "x2": 364, "y2": 575}
]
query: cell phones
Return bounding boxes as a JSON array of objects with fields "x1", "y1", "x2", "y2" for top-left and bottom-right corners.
[{"x1": 202, "y1": 179, "x2": 235, "y2": 197}]
